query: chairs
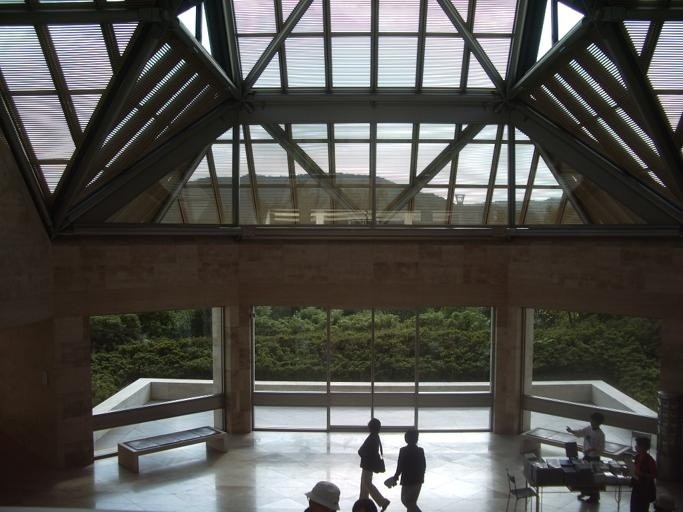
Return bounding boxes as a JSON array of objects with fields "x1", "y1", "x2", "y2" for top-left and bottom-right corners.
[{"x1": 503, "y1": 467, "x2": 538, "y2": 512}]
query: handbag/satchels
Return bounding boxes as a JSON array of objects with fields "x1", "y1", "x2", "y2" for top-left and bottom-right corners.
[{"x1": 374, "y1": 442, "x2": 385, "y2": 472}]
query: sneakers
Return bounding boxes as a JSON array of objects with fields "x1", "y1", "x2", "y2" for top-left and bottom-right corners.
[
  {"x1": 587, "y1": 499, "x2": 599, "y2": 503},
  {"x1": 381, "y1": 499, "x2": 390, "y2": 512},
  {"x1": 578, "y1": 494, "x2": 584, "y2": 498}
]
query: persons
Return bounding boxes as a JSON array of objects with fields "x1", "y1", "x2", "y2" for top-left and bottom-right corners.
[
  {"x1": 566, "y1": 412, "x2": 605, "y2": 503},
  {"x1": 629, "y1": 437, "x2": 657, "y2": 512},
  {"x1": 358, "y1": 418, "x2": 390, "y2": 512},
  {"x1": 352, "y1": 499, "x2": 378, "y2": 512},
  {"x1": 393, "y1": 428, "x2": 426, "y2": 512}
]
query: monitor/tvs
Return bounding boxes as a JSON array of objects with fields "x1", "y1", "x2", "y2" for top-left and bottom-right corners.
[{"x1": 566, "y1": 442, "x2": 577, "y2": 463}]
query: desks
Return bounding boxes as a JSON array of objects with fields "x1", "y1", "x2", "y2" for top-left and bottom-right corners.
[{"x1": 524, "y1": 458, "x2": 634, "y2": 511}]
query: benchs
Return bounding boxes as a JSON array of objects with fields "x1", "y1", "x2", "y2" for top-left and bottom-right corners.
[
  {"x1": 115, "y1": 424, "x2": 230, "y2": 476},
  {"x1": 521, "y1": 426, "x2": 632, "y2": 460}
]
